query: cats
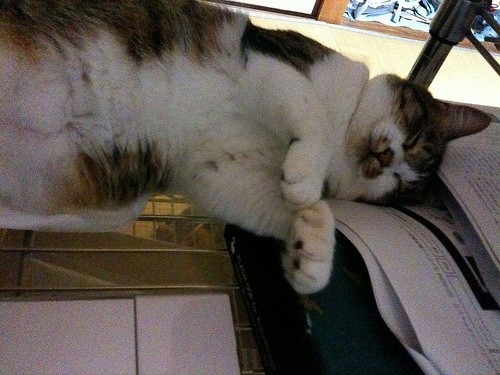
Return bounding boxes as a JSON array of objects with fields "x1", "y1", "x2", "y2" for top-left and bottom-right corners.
[{"x1": 0, "y1": 0, "x2": 493, "y2": 296}]
